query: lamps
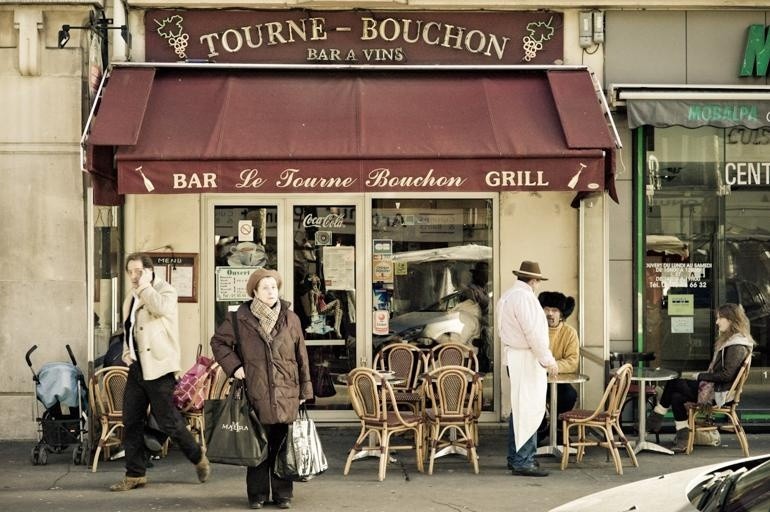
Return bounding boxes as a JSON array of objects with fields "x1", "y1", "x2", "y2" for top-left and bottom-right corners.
[{"x1": 56, "y1": 23, "x2": 134, "y2": 50}]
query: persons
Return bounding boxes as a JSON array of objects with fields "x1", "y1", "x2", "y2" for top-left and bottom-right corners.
[
  {"x1": 112, "y1": 252, "x2": 210, "y2": 491},
  {"x1": 497, "y1": 260, "x2": 557, "y2": 476},
  {"x1": 210, "y1": 268, "x2": 314, "y2": 508},
  {"x1": 304, "y1": 273, "x2": 344, "y2": 338},
  {"x1": 634, "y1": 301, "x2": 758, "y2": 452},
  {"x1": 459, "y1": 261, "x2": 492, "y2": 375},
  {"x1": 535, "y1": 291, "x2": 579, "y2": 445}
]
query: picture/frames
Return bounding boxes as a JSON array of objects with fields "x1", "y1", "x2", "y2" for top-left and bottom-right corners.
[{"x1": 141, "y1": 252, "x2": 200, "y2": 303}]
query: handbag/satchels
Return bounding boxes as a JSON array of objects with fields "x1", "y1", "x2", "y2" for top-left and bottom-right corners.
[{"x1": 173, "y1": 344, "x2": 337, "y2": 482}]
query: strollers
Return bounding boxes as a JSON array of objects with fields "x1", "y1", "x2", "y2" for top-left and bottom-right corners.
[{"x1": 24, "y1": 344, "x2": 89, "y2": 466}]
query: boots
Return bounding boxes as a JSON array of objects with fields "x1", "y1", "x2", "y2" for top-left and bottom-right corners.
[
  {"x1": 634, "y1": 401, "x2": 668, "y2": 432},
  {"x1": 671, "y1": 420, "x2": 690, "y2": 452}
]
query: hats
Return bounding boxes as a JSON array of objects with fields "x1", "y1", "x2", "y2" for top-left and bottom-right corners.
[
  {"x1": 470, "y1": 262, "x2": 488, "y2": 276},
  {"x1": 513, "y1": 261, "x2": 548, "y2": 280},
  {"x1": 538, "y1": 292, "x2": 574, "y2": 319},
  {"x1": 247, "y1": 268, "x2": 281, "y2": 298}
]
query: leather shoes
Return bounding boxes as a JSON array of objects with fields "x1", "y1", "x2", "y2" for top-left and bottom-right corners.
[
  {"x1": 251, "y1": 500, "x2": 264, "y2": 508},
  {"x1": 110, "y1": 475, "x2": 146, "y2": 491},
  {"x1": 508, "y1": 461, "x2": 548, "y2": 475},
  {"x1": 196, "y1": 447, "x2": 209, "y2": 482},
  {"x1": 279, "y1": 497, "x2": 291, "y2": 508}
]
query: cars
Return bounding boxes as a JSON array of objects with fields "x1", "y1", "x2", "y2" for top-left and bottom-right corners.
[
  {"x1": 650, "y1": 281, "x2": 768, "y2": 363},
  {"x1": 373, "y1": 285, "x2": 490, "y2": 373},
  {"x1": 541, "y1": 453, "x2": 770, "y2": 510}
]
